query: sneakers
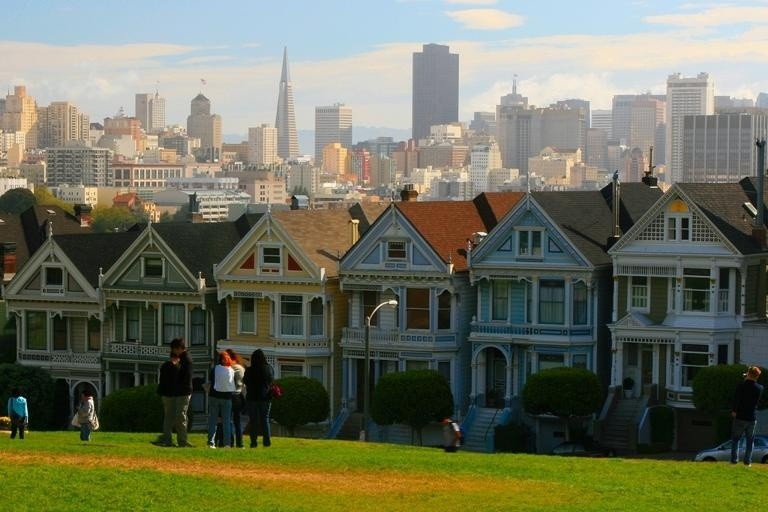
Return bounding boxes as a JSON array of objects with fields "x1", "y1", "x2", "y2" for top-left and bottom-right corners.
[{"x1": 170, "y1": 439, "x2": 272, "y2": 449}]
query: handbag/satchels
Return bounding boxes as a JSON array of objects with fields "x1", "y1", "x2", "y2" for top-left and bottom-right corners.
[
  {"x1": 70, "y1": 411, "x2": 100, "y2": 431},
  {"x1": 10, "y1": 410, "x2": 26, "y2": 428},
  {"x1": 271, "y1": 384, "x2": 282, "y2": 401}
]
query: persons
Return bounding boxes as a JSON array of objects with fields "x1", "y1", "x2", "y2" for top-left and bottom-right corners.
[
  {"x1": 7, "y1": 388, "x2": 29, "y2": 439},
  {"x1": 155, "y1": 351, "x2": 181, "y2": 447},
  {"x1": 437, "y1": 417, "x2": 461, "y2": 453},
  {"x1": 218, "y1": 349, "x2": 248, "y2": 448},
  {"x1": 731, "y1": 366, "x2": 764, "y2": 467},
  {"x1": 243, "y1": 349, "x2": 275, "y2": 447},
  {"x1": 207, "y1": 351, "x2": 236, "y2": 449},
  {"x1": 169, "y1": 338, "x2": 193, "y2": 447},
  {"x1": 76, "y1": 391, "x2": 100, "y2": 442}
]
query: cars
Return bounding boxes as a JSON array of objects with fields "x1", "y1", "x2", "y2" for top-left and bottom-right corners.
[{"x1": 695, "y1": 435, "x2": 768, "y2": 464}]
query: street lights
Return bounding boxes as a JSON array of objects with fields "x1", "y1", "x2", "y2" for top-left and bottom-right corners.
[{"x1": 359, "y1": 299, "x2": 398, "y2": 442}]
